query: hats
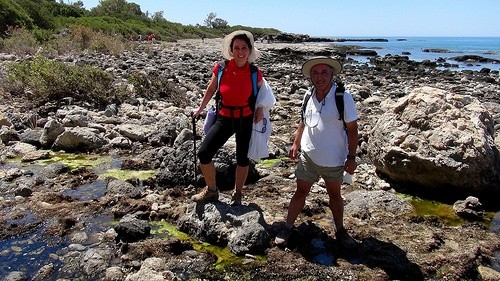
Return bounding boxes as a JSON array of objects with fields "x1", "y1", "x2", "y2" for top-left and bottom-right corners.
[
  {"x1": 301, "y1": 55, "x2": 342, "y2": 79},
  {"x1": 221, "y1": 30, "x2": 260, "y2": 64}
]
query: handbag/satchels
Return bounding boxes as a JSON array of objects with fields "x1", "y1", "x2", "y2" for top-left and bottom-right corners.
[{"x1": 202, "y1": 106, "x2": 216, "y2": 134}]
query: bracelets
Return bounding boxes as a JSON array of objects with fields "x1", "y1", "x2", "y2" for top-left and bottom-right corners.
[{"x1": 347, "y1": 155, "x2": 355, "y2": 159}]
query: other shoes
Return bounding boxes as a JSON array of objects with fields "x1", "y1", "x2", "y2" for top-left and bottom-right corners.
[
  {"x1": 230, "y1": 191, "x2": 244, "y2": 206},
  {"x1": 190, "y1": 185, "x2": 219, "y2": 202},
  {"x1": 274, "y1": 224, "x2": 295, "y2": 245},
  {"x1": 335, "y1": 228, "x2": 356, "y2": 248}
]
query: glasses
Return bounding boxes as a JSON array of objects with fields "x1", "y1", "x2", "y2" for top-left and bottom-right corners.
[{"x1": 252, "y1": 118, "x2": 267, "y2": 133}]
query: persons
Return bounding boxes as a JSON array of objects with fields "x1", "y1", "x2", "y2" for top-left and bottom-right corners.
[
  {"x1": 191, "y1": 30, "x2": 276, "y2": 205},
  {"x1": 148, "y1": 34, "x2": 155, "y2": 44},
  {"x1": 264, "y1": 56, "x2": 358, "y2": 249},
  {"x1": 202, "y1": 35, "x2": 204, "y2": 43}
]
